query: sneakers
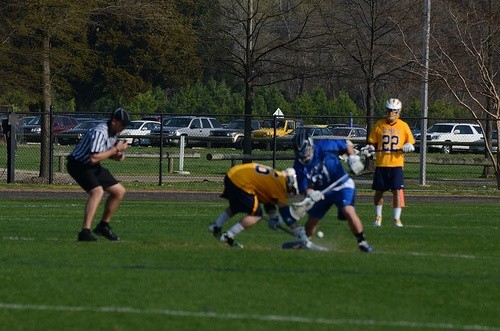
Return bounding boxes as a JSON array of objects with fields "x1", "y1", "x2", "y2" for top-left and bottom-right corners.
[
  {"x1": 374, "y1": 217, "x2": 382, "y2": 226},
  {"x1": 358, "y1": 241, "x2": 372, "y2": 252},
  {"x1": 393, "y1": 219, "x2": 404, "y2": 227},
  {"x1": 283, "y1": 241, "x2": 304, "y2": 249}
]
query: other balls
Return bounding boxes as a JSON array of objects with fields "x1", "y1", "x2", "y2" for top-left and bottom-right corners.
[{"x1": 317, "y1": 231, "x2": 324, "y2": 238}]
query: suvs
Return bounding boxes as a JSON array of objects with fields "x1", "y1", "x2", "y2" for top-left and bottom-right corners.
[
  {"x1": 250, "y1": 118, "x2": 304, "y2": 150},
  {"x1": 149, "y1": 116, "x2": 223, "y2": 148},
  {"x1": 17, "y1": 115, "x2": 79, "y2": 142},
  {"x1": 209, "y1": 119, "x2": 271, "y2": 150}
]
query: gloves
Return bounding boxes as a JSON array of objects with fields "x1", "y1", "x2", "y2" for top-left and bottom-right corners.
[
  {"x1": 362, "y1": 144, "x2": 375, "y2": 157},
  {"x1": 403, "y1": 143, "x2": 414, "y2": 152},
  {"x1": 307, "y1": 189, "x2": 324, "y2": 202},
  {"x1": 347, "y1": 154, "x2": 365, "y2": 174},
  {"x1": 292, "y1": 225, "x2": 310, "y2": 245},
  {"x1": 269, "y1": 216, "x2": 280, "y2": 229}
]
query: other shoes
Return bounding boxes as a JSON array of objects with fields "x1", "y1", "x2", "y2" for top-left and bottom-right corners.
[
  {"x1": 93, "y1": 222, "x2": 120, "y2": 241},
  {"x1": 219, "y1": 233, "x2": 244, "y2": 248},
  {"x1": 208, "y1": 224, "x2": 223, "y2": 237},
  {"x1": 78, "y1": 231, "x2": 98, "y2": 241}
]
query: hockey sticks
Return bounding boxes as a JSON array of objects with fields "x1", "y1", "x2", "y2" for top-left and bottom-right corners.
[
  {"x1": 261, "y1": 215, "x2": 328, "y2": 251},
  {"x1": 339, "y1": 147, "x2": 404, "y2": 160},
  {"x1": 289, "y1": 154, "x2": 374, "y2": 220}
]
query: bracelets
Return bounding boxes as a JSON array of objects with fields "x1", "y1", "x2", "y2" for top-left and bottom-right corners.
[{"x1": 115, "y1": 146, "x2": 118, "y2": 153}]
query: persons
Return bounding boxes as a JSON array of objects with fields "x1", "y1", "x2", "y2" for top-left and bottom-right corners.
[
  {"x1": 361, "y1": 97, "x2": 415, "y2": 227},
  {"x1": 66, "y1": 108, "x2": 131, "y2": 241},
  {"x1": 208, "y1": 163, "x2": 311, "y2": 248},
  {"x1": 282, "y1": 131, "x2": 374, "y2": 252}
]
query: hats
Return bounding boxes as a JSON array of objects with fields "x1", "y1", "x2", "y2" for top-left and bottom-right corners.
[{"x1": 111, "y1": 108, "x2": 135, "y2": 127}]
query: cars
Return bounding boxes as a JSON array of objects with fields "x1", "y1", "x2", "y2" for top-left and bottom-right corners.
[
  {"x1": 323, "y1": 128, "x2": 367, "y2": 138},
  {"x1": 469, "y1": 129, "x2": 498, "y2": 154},
  {"x1": 117, "y1": 120, "x2": 161, "y2": 146},
  {"x1": 414, "y1": 123, "x2": 484, "y2": 154},
  {"x1": 280, "y1": 127, "x2": 333, "y2": 148},
  {"x1": 57, "y1": 120, "x2": 108, "y2": 145}
]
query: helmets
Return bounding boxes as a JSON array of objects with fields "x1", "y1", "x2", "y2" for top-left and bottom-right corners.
[
  {"x1": 385, "y1": 98, "x2": 402, "y2": 122},
  {"x1": 292, "y1": 133, "x2": 314, "y2": 160},
  {"x1": 285, "y1": 168, "x2": 300, "y2": 195}
]
